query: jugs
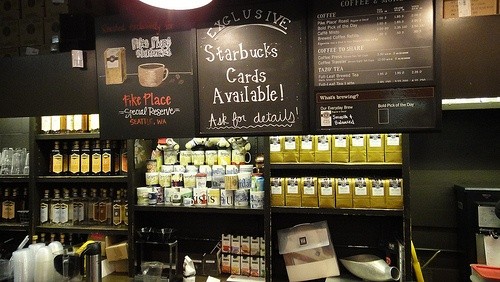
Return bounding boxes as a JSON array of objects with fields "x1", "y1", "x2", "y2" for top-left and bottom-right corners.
[{"x1": 79, "y1": 242, "x2": 103, "y2": 282}]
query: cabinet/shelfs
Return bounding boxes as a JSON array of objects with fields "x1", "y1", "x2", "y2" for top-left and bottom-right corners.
[
  {"x1": 34, "y1": 115, "x2": 129, "y2": 259},
  {"x1": 131, "y1": 131, "x2": 268, "y2": 282},
  {"x1": 270, "y1": 131, "x2": 411, "y2": 282},
  {"x1": 0, "y1": 115, "x2": 31, "y2": 232}
]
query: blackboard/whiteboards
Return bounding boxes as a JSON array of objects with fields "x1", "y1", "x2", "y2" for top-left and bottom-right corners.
[
  {"x1": 95, "y1": 26, "x2": 197, "y2": 138},
  {"x1": 196, "y1": 6, "x2": 311, "y2": 135}
]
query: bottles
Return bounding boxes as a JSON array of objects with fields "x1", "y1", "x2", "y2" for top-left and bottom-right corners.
[
  {"x1": 0, "y1": 147, "x2": 30, "y2": 175},
  {"x1": 0, "y1": 187, "x2": 30, "y2": 226},
  {"x1": 49, "y1": 140, "x2": 129, "y2": 176},
  {"x1": 33, "y1": 232, "x2": 116, "y2": 282},
  {"x1": 38, "y1": 185, "x2": 129, "y2": 229}
]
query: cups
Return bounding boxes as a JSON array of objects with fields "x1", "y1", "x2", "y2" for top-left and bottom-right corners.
[
  {"x1": 40, "y1": 113, "x2": 100, "y2": 134},
  {"x1": 136, "y1": 137, "x2": 265, "y2": 209},
  {"x1": 133, "y1": 261, "x2": 170, "y2": 282}
]
